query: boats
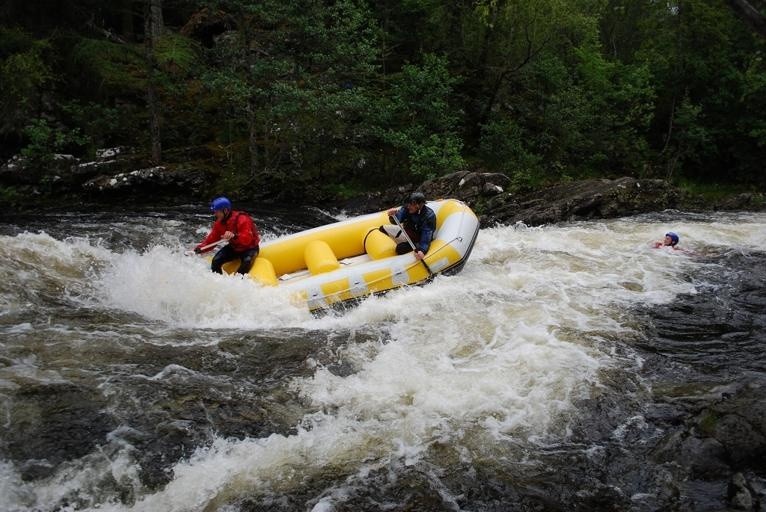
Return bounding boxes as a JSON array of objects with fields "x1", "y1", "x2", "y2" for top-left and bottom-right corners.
[{"x1": 204, "y1": 198, "x2": 482, "y2": 318}]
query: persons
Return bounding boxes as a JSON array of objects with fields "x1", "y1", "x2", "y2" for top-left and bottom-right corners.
[
  {"x1": 380, "y1": 190, "x2": 437, "y2": 260},
  {"x1": 653, "y1": 229, "x2": 699, "y2": 258},
  {"x1": 189, "y1": 197, "x2": 260, "y2": 276}
]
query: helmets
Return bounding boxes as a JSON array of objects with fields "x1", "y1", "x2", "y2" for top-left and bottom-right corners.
[
  {"x1": 666, "y1": 232, "x2": 679, "y2": 243},
  {"x1": 211, "y1": 197, "x2": 229, "y2": 210},
  {"x1": 407, "y1": 193, "x2": 426, "y2": 205}
]
